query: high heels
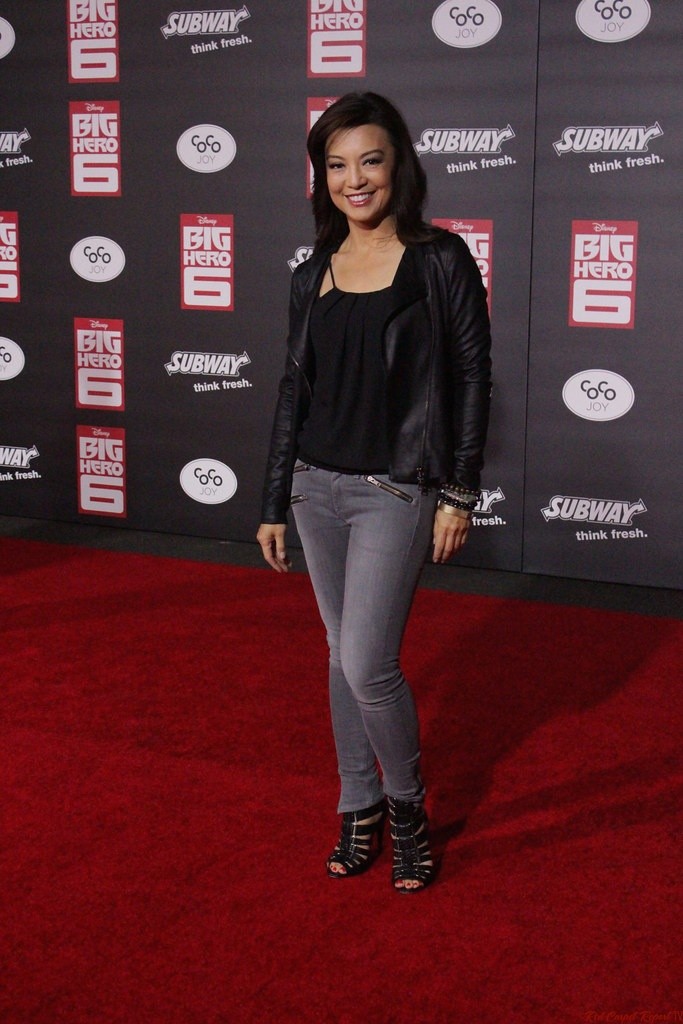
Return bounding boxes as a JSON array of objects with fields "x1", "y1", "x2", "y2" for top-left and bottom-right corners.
[
  {"x1": 326, "y1": 797, "x2": 387, "y2": 877},
  {"x1": 385, "y1": 794, "x2": 434, "y2": 893}
]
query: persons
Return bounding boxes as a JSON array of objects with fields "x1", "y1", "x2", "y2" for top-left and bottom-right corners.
[{"x1": 255, "y1": 91, "x2": 493, "y2": 894}]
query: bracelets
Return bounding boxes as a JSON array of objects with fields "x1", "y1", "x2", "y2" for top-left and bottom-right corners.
[{"x1": 436, "y1": 485, "x2": 480, "y2": 520}]
